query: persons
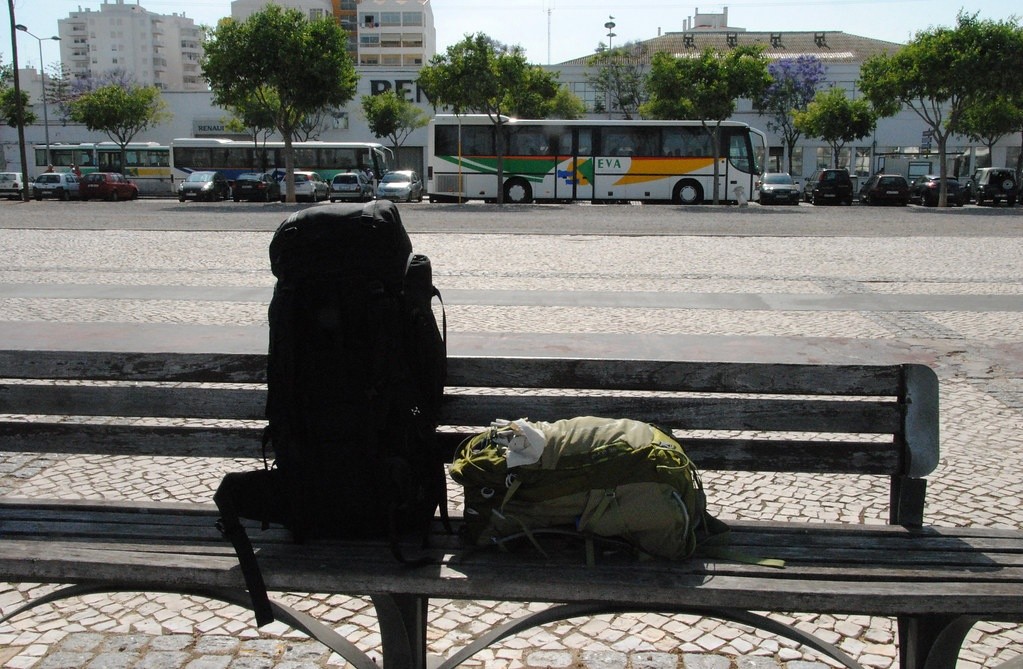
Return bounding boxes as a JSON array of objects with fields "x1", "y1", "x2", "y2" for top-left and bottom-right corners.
[
  {"x1": 43, "y1": 164, "x2": 55, "y2": 173},
  {"x1": 69, "y1": 163, "x2": 81, "y2": 178}
]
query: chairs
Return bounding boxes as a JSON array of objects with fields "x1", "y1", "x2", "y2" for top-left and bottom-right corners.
[{"x1": 609, "y1": 146, "x2": 710, "y2": 157}]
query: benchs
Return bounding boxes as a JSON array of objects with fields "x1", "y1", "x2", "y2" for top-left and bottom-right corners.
[{"x1": 0, "y1": 348, "x2": 1023, "y2": 669}]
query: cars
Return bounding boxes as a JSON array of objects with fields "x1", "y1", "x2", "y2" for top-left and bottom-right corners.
[
  {"x1": 281, "y1": 170, "x2": 330, "y2": 202},
  {"x1": 34, "y1": 173, "x2": 82, "y2": 201},
  {"x1": 79, "y1": 173, "x2": 138, "y2": 202},
  {"x1": 330, "y1": 174, "x2": 375, "y2": 201},
  {"x1": 0, "y1": 172, "x2": 36, "y2": 201},
  {"x1": 859, "y1": 173, "x2": 909, "y2": 207},
  {"x1": 377, "y1": 169, "x2": 424, "y2": 203},
  {"x1": 758, "y1": 171, "x2": 800, "y2": 206},
  {"x1": 233, "y1": 172, "x2": 280, "y2": 201},
  {"x1": 177, "y1": 171, "x2": 230, "y2": 201},
  {"x1": 802, "y1": 169, "x2": 853, "y2": 205},
  {"x1": 909, "y1": 174, "x2": 965, "y2": 206}
]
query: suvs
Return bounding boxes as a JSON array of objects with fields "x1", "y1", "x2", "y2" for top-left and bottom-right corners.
[{"x1": 966, "y1": 166, "x2": 1018, "y2": 206}]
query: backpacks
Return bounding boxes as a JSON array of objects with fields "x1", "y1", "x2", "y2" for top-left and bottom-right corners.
[
  {"x1": 446, "y1": 418, "x2": 712, "y2": 574},
  {"x1": 216, "y1": 198, "x2": 454, "y2": 549}
]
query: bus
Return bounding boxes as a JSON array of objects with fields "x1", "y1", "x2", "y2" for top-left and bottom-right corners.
[
  {"x1": 32, "y1": 141, "x2": 171, "y2": 194},
  {"x1": 428, "y1": 113, "x2": 767, "y2": 206},
  {"x1": 169, "y1": 136, "x2": 393, "y2": 191}
]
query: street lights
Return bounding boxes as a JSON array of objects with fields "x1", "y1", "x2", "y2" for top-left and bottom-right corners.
[
  {"x1": 605, "y1": 21, "x2": 615, "y2": 120},
  {"x1": 15, "y1": 23, "x2": 61, "y2": 166}
]
